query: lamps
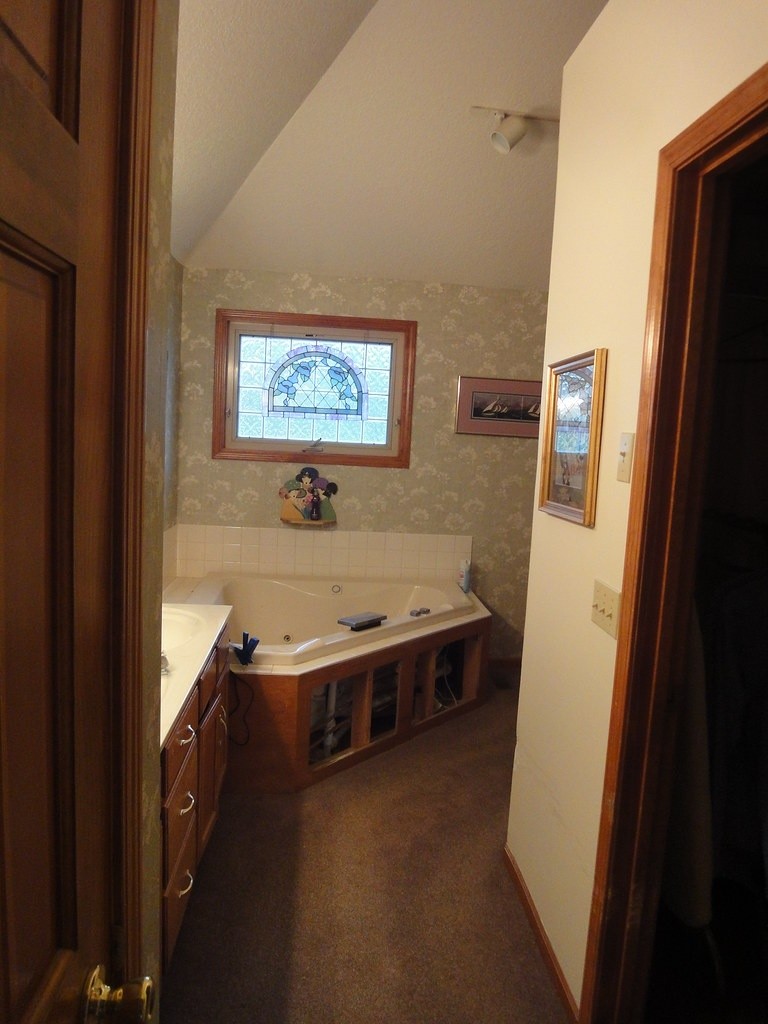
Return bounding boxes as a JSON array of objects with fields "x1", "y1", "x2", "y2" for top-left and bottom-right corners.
[{"x1": 488, "y1": 110, "x2": 529, "y2": 155}]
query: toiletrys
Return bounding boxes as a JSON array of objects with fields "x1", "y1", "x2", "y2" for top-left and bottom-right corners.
[{"x1": 459, "y1": 558, "x2": 472, "y2": 593}]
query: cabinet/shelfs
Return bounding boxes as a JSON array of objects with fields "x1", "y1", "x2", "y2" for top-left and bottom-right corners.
[{"x1": 161, "y1": 622, "x2": 231, "y2": 967}]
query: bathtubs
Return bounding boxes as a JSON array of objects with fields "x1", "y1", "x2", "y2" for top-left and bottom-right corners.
[{"x1": 185, "y1": 569, "x2": 493, "y2": 793}]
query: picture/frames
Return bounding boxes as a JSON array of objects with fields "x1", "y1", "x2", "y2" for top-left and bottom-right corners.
[
  {"x1": 455, "y1": 374, "x2": 543, "y2": 438},
  {"x1": 538, "y1": 347, "x2": 608, "y2": 529}
]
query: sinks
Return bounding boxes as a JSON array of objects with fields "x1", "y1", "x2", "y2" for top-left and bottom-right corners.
[{"x1": 162, "y1": 609, "x2": 210, "y2": 713}]
